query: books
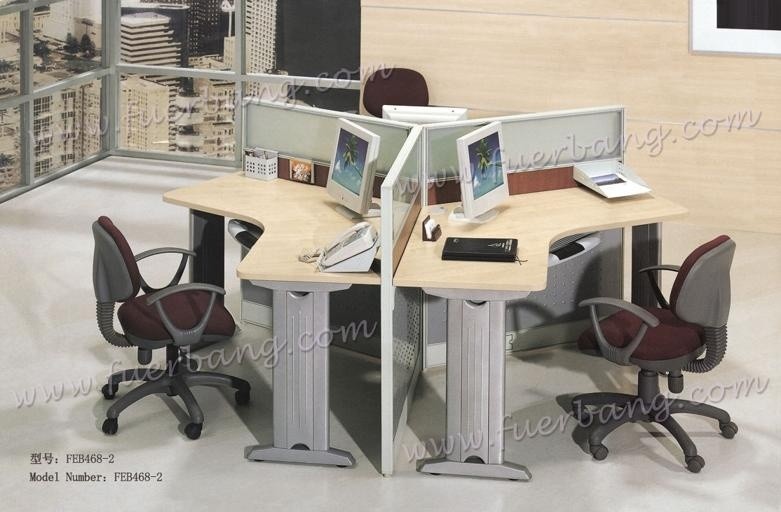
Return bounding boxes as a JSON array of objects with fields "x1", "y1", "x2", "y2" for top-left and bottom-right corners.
[{"x1": 441, "y1": 236, "x2": 518, "y2": 263}]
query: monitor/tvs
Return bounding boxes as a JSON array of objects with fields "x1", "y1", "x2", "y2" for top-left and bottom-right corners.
[
  {"x1": 381, "y1": 104, "x2": 467, "y2": 124},
  {"x1": 451, "y1": 121, "x2": 509, "y2": 224},
  {"x1": 326, "y1": 117, "x2": 381, "y2": 220}
]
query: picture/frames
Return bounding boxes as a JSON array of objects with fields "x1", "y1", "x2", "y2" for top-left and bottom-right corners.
[{"x1": 688, "y1": 0, "x2": 781, "y2": 56}]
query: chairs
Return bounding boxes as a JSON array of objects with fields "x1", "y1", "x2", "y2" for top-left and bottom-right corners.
[
  {"x1": 573, "y1": 235, "x2": 739, "y2": 476},
  {"x1": 90, "y1": 215, "x2": 250, "y2": 439},
  {"x1": 362, "y1": 67, "x2": 429, "y2": 117}
]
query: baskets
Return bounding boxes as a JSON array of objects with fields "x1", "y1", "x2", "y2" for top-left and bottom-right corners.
[{"x1": 245, "y1": 147, "x2": 278, "y2": 180}]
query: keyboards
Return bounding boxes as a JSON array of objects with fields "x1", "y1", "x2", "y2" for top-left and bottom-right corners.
[{"x1": 550, "y1": 232, "x2": 590, "y2": 253}]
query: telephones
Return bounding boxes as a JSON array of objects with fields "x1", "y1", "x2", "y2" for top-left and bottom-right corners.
[{"x1": 321, "y1": 222, "x2": 379, "y2": 267}]
